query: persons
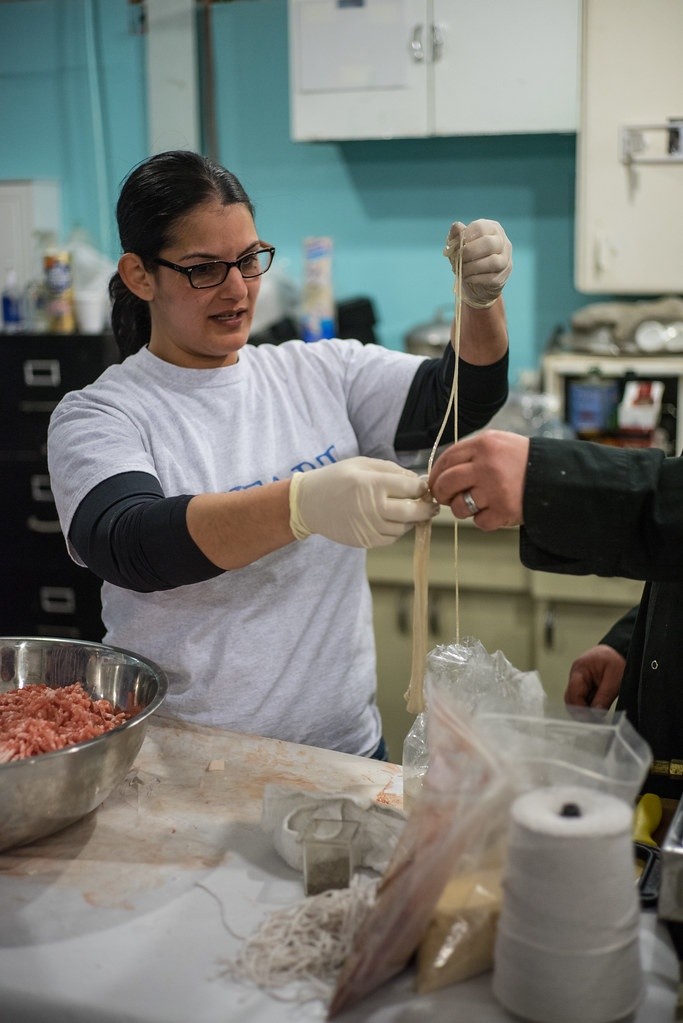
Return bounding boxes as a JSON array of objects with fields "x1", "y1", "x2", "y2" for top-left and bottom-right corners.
[
  {"x1": 429, "y1": 429, "x2": 683, "y2": 803},
  {"x1": 48, "y1": 149, "x2": 514, "y2": 761}
]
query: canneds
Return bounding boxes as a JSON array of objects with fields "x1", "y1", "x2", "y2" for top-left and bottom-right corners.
[{"x1": 43, "y1": 251, "x2": 76, "y2": 335}]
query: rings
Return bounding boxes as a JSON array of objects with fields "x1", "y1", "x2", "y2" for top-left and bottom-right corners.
[{"x1": 464, "y1": 492, "x2": 480, "y2": 516}]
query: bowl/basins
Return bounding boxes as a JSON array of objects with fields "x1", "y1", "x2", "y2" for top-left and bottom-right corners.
[{"x1": 0, "y1": 637, "x2": 168, "y2": 851}]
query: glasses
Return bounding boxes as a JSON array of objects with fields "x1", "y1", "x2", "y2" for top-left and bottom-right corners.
[{"x1": 145, "y1": 241, "x2": 275, "y2": 289}]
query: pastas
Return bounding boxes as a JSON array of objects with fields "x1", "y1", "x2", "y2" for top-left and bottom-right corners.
[
  {"x1": 403, "y1": 230, "x2": 463, "y2": 712},
  {"x1": 194, "y1": 872, "x2": 382, "y2": 1010}
]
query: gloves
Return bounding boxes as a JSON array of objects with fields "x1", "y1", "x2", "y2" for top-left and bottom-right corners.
[
  {"x1": 443, "y1": 219, "x2": 514, "y2": 309},
  {"x1": 288, "y1": 455, "x2": 440, "y2": 550}
]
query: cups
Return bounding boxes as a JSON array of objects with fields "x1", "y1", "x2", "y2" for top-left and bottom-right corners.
[
  {"x1": 302, "y1": 820, "x2": 362, "y2": 896},
  {"x1": 74, "y1": 300, "x2": 103, "y2": 335}
]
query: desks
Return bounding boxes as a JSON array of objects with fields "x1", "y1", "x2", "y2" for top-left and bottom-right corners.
[{"x1": 0, "y1": 714, "x2": 683, "y2": 1023}]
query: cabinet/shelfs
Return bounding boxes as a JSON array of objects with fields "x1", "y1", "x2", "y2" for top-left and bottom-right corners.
[
  {"x1": 0, "y1": 332, "x2": 123, "y2": 644},
  {"x1": 362, "y1": 502, "x2": 648, "y2": 767},
  {"x1": 286, "y1": 0, "x2": 583, "y2": 144},
  {"x1": 575, "y1": 0, "x2": 683, "y2": 300}
]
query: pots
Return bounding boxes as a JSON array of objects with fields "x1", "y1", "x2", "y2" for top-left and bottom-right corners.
[{"x1": 404, "y1": 307, "x2": 457, "y2": 358}]
